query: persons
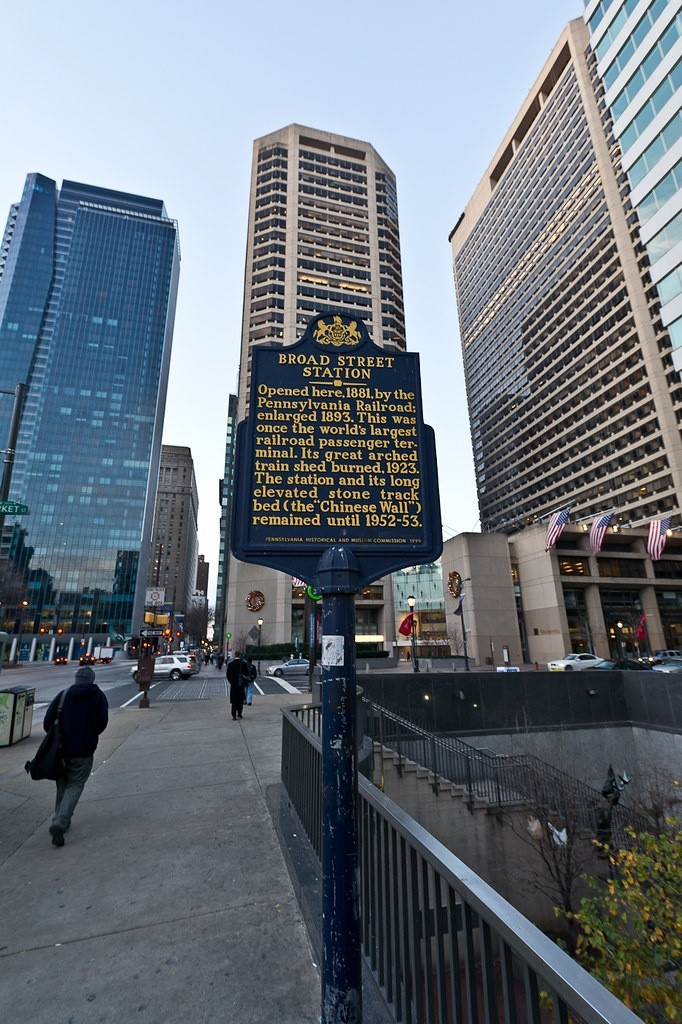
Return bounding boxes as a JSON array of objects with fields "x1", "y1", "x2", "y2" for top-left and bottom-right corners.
[
  {"x1": 44, "y1": 667, "x2": 109, "y2": 846},
  {"x1": 243, "y1": 657, "x2": 257, "y2": 706},
  {"x1": 283, "y1": 655, "x2": 290, "y2": 663},
  {"x1": 204, "y1": 649, "x2": 225, "y2": 671},
  {"x1": 594, "y1": 807, "x2": 612, "y2": 843},
  {"x1": 226, "y1": 651, "x2": 250, "y2": 720},
  {"x1": 406, "y1": 651, "x2": 411, "y2": 662}
]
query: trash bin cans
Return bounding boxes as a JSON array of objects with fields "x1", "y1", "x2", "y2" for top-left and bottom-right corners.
[{"x1": 0, "y1": 685, "x2": 35, "y2": 747}]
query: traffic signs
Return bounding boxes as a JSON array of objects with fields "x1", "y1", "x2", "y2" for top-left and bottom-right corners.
[{"x1": 139, "y1": 628, "x2": 164, "y2": 637}]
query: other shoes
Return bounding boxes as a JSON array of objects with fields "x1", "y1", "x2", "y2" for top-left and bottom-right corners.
[
  {"x1": 244, "y1": 702, "x2": 247, "y2": 704},
  {"x1": 248, "y1": 702, "x2": 251, "y2": 706},
  {"x1": 49, "y1": 825, "x2": 67, "y2": 846},
  {"x1": 232, "y1": 716, "x2": 237, "y2": 721},
  {"x1": 237, "y1": 714, "x2": 242, "y2": 718}
]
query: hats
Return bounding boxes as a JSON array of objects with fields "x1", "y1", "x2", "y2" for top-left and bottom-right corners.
[{"x1": 75, "y1": 666, "x2": 95, "y2": 684}]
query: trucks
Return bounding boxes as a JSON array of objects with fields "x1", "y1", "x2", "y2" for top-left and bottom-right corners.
[{"x1": 93, "y1": 647, "x2": 114, "y2": 664}]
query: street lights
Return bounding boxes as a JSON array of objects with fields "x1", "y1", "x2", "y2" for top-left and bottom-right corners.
[
  {"x1": 407, "y1": 595, "x2": 421, "y2": 672},
  {"x1": 257, "y1": 616, "x2": 262, "y2": 675},
  {"x1": 617, "y1": 620, "x2": 626, "y2": 656}
]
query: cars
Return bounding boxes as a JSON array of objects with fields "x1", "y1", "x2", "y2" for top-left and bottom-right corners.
[
  {"x1": 53, "y1": 657, "x2": 67, "y2": 665},
  {"x1": 172, "y1": 644, "x2": 211, "y2": 663},
  {"x1": 640, "y1": 649, "x2": 682, "y2": 675},
  {"x1": 581, "y1": 656, "x2": 652, "y2": 671},
  {"x1": 266, "y1": 659, "x2": 322, "y2": 678},
  {"x1": 547, "y1": 652, "x2": 607, "y2": 672},
  {"x1": 78, "y1": 654, "x2": 95, "y2": 665}
]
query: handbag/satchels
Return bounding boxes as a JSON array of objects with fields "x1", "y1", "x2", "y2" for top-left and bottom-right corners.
[
  {"x1": 239, "y1": 674, "x2": 249, "y2": 687},
  {"x1": 29, "y1": 722, "x2": 67, "y2": 781}
]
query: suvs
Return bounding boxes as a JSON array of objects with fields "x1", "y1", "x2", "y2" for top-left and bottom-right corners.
[{"x1": 129, "y1": 653, "x2": 200, "y2": 684}]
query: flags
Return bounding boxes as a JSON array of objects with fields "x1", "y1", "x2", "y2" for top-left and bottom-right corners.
[
  {"x1": 544, "y1": 508, "x2": 571, "y2": 552},
  {"x1": 647, "y1": 516, "x2": 671, "y2": 560},
  {"x1": 590, "y1": 512, "x2": 615, "y2": 556},
  {"x1": 636, "y1": 615, "x2": 647, "y2": 640}
]
